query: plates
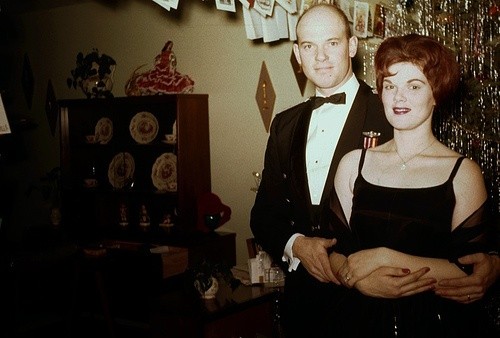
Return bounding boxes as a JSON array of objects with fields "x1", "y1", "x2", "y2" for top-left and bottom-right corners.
[
  {"x1": 163, "y1": 140, "x2": 176, "y2": 144},
  {"x1": 151, "y1": 152, "x2": 178, "y2": 192},
  {"x1": 129, "y1": 111, "x2": 159, "y2": 144},
  {"x1": 107, "y1": 152, "x2": 135, "y2": 189},
  {"x1": 85, "y1": 140, "x2": 100, "y2": 144},
  {"x1": 83, "y1": 184, "x2": 100, "y2": 187},
  {"x1": 94, "y1": 117, "x2": 113, "y2": 144}
]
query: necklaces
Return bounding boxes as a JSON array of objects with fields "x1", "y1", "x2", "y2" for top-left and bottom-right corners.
[{"x1": 392, "y1": 137, "x2": 437, "y2": 168}]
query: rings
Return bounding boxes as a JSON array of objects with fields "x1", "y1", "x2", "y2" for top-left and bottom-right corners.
[
  {"x1": 468, "y1": 295, "x2": 471, "y2": 301},
  {"x1": 347, "y1": 277, "x2": 350, "y2": 280}
]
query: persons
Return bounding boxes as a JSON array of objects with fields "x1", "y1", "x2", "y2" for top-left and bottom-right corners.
[
  {"x1": 322, "y1": 33, "x2": 492, "y2": 338},
  {"x1": 249, "y1": 2, "x2": 500, "y2": 338}
]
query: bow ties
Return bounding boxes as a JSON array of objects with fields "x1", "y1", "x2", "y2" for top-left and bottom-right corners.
[{"x1": 309, "y1": 92, "x2": 346, "y2": 109}]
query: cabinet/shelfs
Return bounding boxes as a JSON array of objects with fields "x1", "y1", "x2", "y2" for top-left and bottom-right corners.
[
  {"x1": 32, "y1": 229, "x2": 281, "y2": 338},
  {"x1": 54, "y1": 93, "x2": 212, "y2": 236}
]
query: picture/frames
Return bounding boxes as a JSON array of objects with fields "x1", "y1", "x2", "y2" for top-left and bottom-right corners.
[{"x1": 352, "y1": 1, "x2": 369, "y2": 39}]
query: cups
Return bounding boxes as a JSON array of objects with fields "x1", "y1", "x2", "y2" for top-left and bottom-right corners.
[
  {"x1": 87, "y1": 135, "x2": 96, "y2": 142},
  {"x1": 85, "y1": 179, "x2": 97, "y2": 185},
  {"x1": 258, "y1": 251, "x2": 283, "y2": 288},
  {"x1": 167, "y1": 182, "x2": 177, "y2": 192},
  {"x1": 164, "y1": 134, "x2": 176, "y2": 141}
]
